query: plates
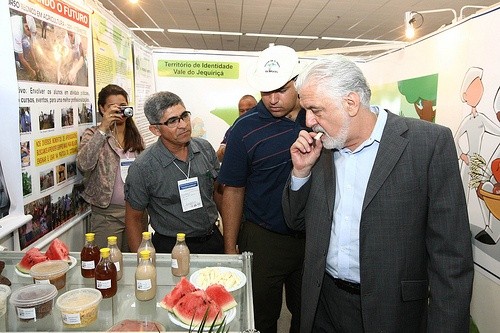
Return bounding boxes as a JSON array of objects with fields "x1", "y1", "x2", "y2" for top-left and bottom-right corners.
[
  {"x1": 171, "y1": 310, "x2": 237, "y2": 331},
  {"x1": 15, "y1": 255, "x2": 77, "y2": 277},
  {"x1": 189, "y1": 266, "x2": 247, "y2": 292}
]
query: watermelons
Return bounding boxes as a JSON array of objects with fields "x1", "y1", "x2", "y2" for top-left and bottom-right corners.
[
  {"x1": 158, "y1": 276, "x2": 237, "y2": 327},
  {"x1": 16, "y1": 238, "x2": 69, "y2": 274}
]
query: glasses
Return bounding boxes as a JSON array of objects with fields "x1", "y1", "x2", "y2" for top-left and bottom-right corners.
[{"x1": 151, "y1": 111, "x2": 192, "y2": 128}]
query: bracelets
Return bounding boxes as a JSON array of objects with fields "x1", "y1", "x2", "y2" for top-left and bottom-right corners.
[{"x1": 97, "y1": 129, "x2": 107, "y2": 136}]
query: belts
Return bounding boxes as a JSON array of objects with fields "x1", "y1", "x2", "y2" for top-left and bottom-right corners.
[
  {"x1": 186, "y1": 228, "x2": 216, "y2": 243},
  {"x1": 328, "y1": 272, "x2": 360, "y2": 295}
]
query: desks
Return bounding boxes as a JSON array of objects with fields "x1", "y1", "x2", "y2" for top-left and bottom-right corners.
[{"x1": 0, "y1": 250, "x2": 256, "y2": 332}]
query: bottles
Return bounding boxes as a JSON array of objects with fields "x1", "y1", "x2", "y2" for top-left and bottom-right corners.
[
  {"x1": 136, "y1": 232, "x2": 156, "y2": 268},
  {"x1": 135, "y1": 251, "x2": 156, "y2": 301},
  {"x1": 95, "y1": 248, "x2": 118, "y2": 299},
  {"x1": 107, "y1": 236, "x2": 123, "y2": 281},
  {"x1": 171, "y1": 234, "x2": 190, "y2": 277},
  {"x1": 81, "y1": 233, "x2": 100, "y2": 278}
]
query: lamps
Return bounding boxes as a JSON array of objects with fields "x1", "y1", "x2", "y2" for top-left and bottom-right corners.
[{"x1": 405, "y1": 8, "x2": 456, "y2": 39}]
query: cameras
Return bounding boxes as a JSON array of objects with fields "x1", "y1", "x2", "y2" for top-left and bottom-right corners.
[{"x1": 115, "y1": 106, "x2": 133, "y2": 117}]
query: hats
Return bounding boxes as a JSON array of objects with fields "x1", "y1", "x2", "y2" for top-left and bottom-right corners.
[{"x1": 256, "y1": 45, "x2": 300, "y2": 93}]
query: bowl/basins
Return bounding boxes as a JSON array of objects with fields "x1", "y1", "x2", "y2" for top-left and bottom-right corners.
[
  {"x1": 30, "y1": 260, "x2": 70, "y2": 289},
  {"x1": 55, "y1": 288, "x2": 103, "y2": 328},
  {"x1": 0, "y1": 284, "x2": 12, "y2": 318},
  {"x1": 10, "y1": 283, "x2": 58, "y2": 323}
]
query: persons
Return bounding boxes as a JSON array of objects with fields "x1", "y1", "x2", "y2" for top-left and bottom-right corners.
[
  {"x1": 282, "y1": 56, "x2": 475, "y2": 333},
  {"x1": 219, "y1": 45, "x2": 313, "y2": 333},
  {"x1": 19, "y1": 104, "x2": 94, "y2": 246},
  {"x1": 41, "y1": 21, "x2": 47, "y2": 40},
  {"x1": 11, "y1": 15, "x2": 37, "y2": 78},
  {"x1": 124, "y1": 91, "x2": 225, "y2": 254},
  {"x1": 77, "y1": 84, "x2": 144, "y2": 253},
  {"x1": 217, "y1": 95, "x2": 257, "y2": 163},
  {"x1": 57, "y1": 30, "x2": 86, "y2": 85}
]
